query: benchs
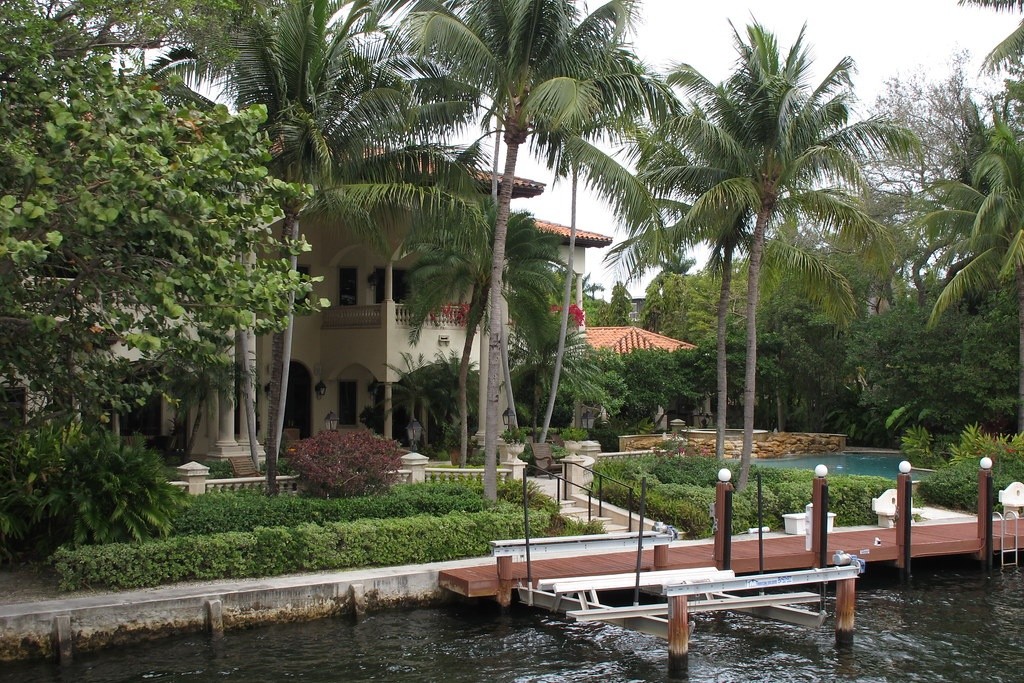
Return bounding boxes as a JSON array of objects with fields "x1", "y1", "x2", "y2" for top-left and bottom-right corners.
[
  {"x1": 781, "y1": 513, "x2": 837, "y2": 535},
  {"x1": 999, "y1": 481, "x2": 1024, "y2": 519},
  {"x1": 872, "y1": 489, "x2": 925, "y2": 528}
]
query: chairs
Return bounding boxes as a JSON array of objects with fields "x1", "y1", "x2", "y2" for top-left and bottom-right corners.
[
  {"x1": 530, "y1": 442, "x2": 562, "y2": 480},
  {"x1": 228, "y1": 456, "x2": 260, "y2": 478}
]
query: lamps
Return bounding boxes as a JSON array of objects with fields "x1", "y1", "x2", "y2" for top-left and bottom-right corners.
[
  {"x1": 264, "y1": 382, "x2": 270, "y2": 399},
  {"x1": 324, "y1": 411, "x2": 340, "y2": 432},
  {"x1": 315, "y1": 380, "x2": 327, "y2": 400},
  {"x1": 368, "y1": 271, "x2": 378, "y2": 286},
  {"x1": 406, "y1": 418, "x2": 423, "y2": 451},
  {"x1": 502, "y1": 409, "x2": 516, "y2": 429}
]
